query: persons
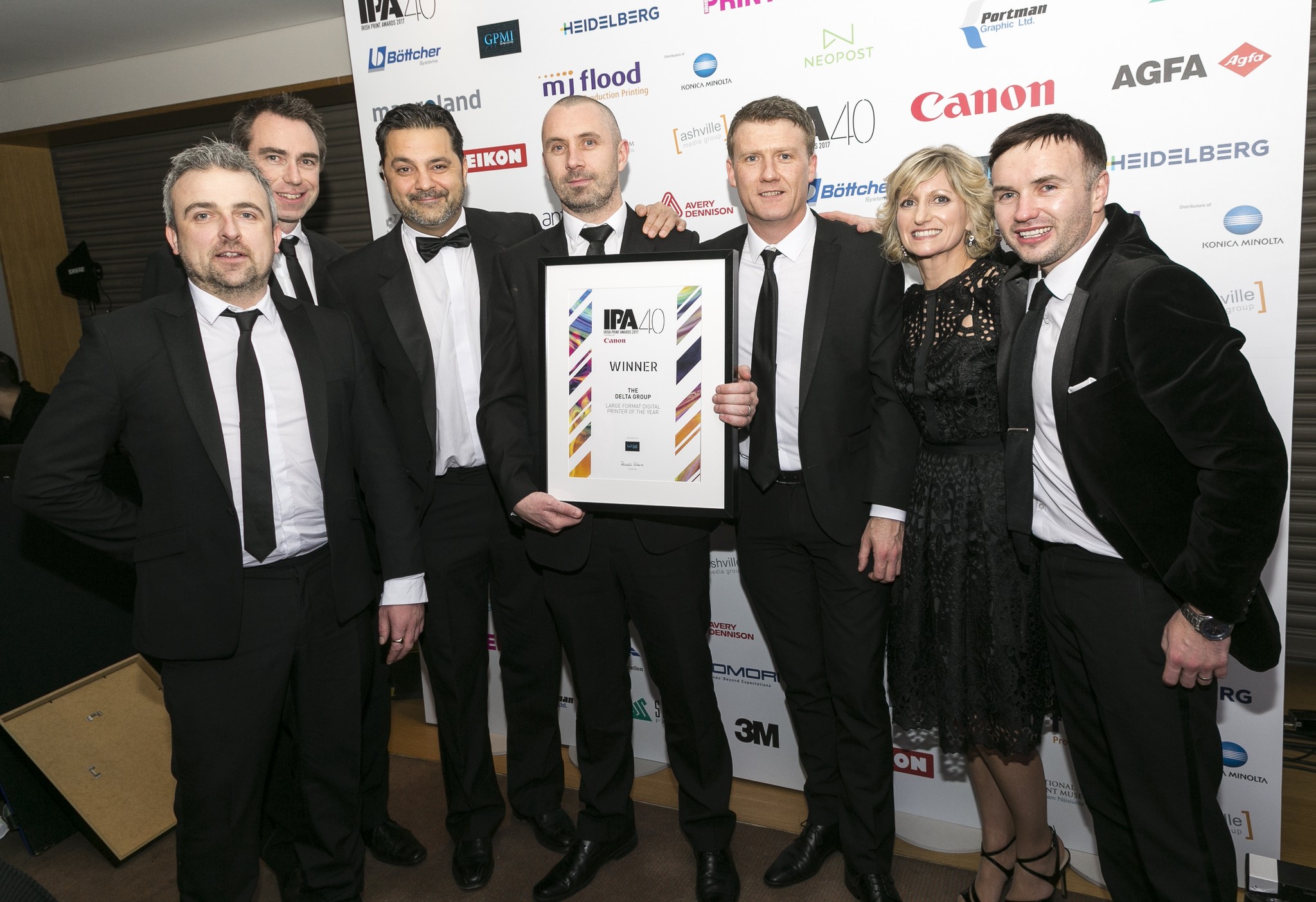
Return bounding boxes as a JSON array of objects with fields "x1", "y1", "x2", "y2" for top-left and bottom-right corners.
[
  {"x1": 880, "y1": 143, "x2": 1079, "y2": 902},
  {"x1": 0, "y1": 351, "x2": 67, "y2": 445},
  {"x1": 821, "y1": 111, "x2": 1291, "y2": 901},
  {"x1": 9, "y1": 137, "x2": 430, "y2": 900},
  {"x1": 695, "y1": 95, "x2": 911, "y2": 902},
  {"x1": 328, "y1": 102, "x2": 688, "y2": 895},
  {"x1": 474, "y1": 95, "x2": 760, "y2": 902},
  {"x1": 228, "y1": 89, "x2": 431, "y2": 884}
]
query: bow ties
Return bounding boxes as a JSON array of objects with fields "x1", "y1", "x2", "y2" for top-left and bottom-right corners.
[{"x1": 415, "y1": 225, "x2": 471, "y2": 263}]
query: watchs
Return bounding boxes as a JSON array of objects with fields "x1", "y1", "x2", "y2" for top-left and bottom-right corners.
[{"x1": 1179, "y1": 600, "x2": 1237, "y2": 640}]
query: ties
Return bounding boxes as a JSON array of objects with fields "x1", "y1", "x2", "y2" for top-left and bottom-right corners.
[
  {"x1": 279, "y1": 235, "x2": 315, "y2": 307},
  {"x1": 218, "y1": 307, "x2": 278, "y2": 564},
  {"x1": 579, "y1": 224, "x2": 614, "y2": 256},
  {"x1": 748, "y1": 250, "x2": 781, "y2": 492},
  {"x1": 1006, "y1": 278, "x2": 1052, "y2": 569}
]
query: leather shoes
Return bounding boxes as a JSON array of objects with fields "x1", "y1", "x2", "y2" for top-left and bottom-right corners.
[
  {"x1": 533, "y1": 833, "x2": 639, "y2": 902},
  {"x1": 845, "y1": 864, "x2": 902, "y2": 902},
  {"x1": 763, "y1": 821, "x2": 842, "y2": 886},
  {"x1": 693, "y1": 846, "x2": 740, "y2": 902},
  {"x1": 453, "y1": 838, "x2": 494, "y2": 889},
  {"x1": 513, "y1": 807, "x2": 579, "y2": 853},
  {"x1": 364, "y1": 821, "x2": 428, "y2": 866}
]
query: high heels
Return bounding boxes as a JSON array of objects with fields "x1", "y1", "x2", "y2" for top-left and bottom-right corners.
[
  {"x1": 1003, "y1": 826, "x2": 1071, "y2": 902},
  {"x1": 960, "y1": 837, "x2": 1015, "y2": 902}
]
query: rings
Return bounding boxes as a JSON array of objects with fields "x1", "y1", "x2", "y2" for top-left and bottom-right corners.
[
  {"x1": 746, "y1": 405, "x2": 751, "y2": 417},
  {"x1": 391, "y1": 637, "x2": 404, "y2": 646}
]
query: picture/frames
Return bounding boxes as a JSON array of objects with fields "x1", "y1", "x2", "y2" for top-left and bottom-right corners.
[{"x1": 537, "y1": 248, "x2": 739, "y2": 520}]
